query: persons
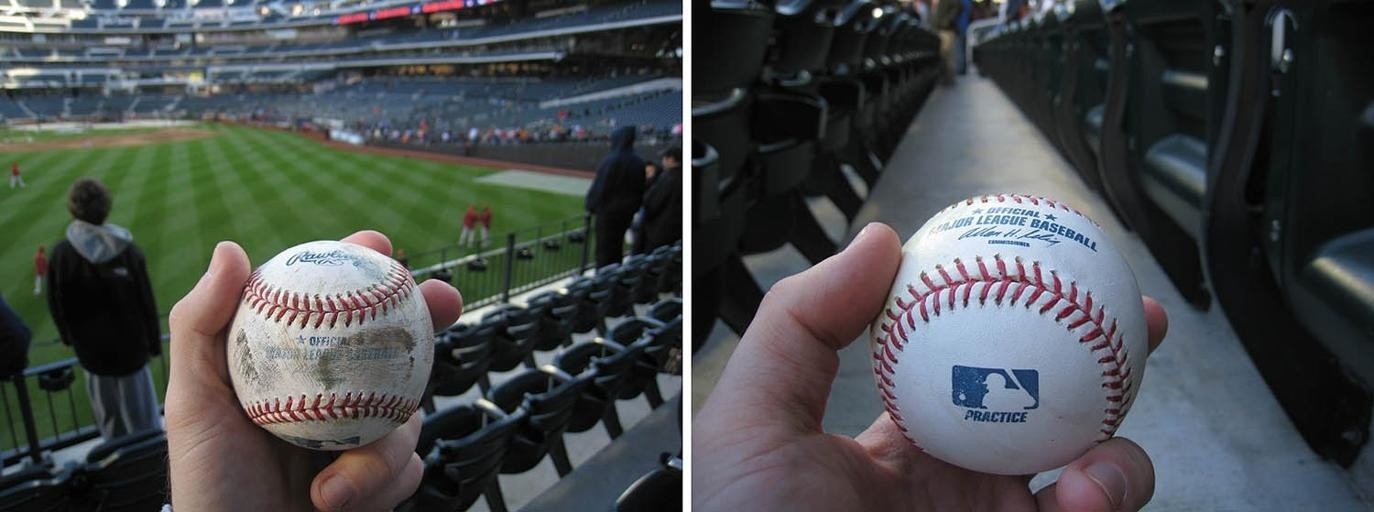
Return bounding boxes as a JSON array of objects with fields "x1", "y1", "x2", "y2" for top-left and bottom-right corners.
[
  {"x1": 457, "y1": 204, "x2": 478, "y2": 249},
  {"x1": 480, "y1": 205, "x2": 492, "y2": 248},
  {"x1": 167, "y1": 228, "x2": 464, "y2": 511},
  {"x1": 632, "y1": 162, "x2": 658, "y2": 232},
  {"x1": 32, "y1": 245, "x2": 49, "y2": 295},
  {"x1": 45, "y1": 178, "x2": 163, "y2": 444},
  {"x1": 586, "y1": 125, "x2": 646, "y2": 274},
  {"x1": 692, "y1": 222, "x2": 1170, "y2": 509},
  {"x1": 631, "y1": 146, "x2": 680, "y2": 255},
  {"x1": 394, "y1": 246, "x2": 408, "y2": 270},
  {"x1": 952, "y1": 0, "x2": 974, "y2": 75},
  {"x1": 10, "y1": 159, "x2": 26, "y2": 190}
]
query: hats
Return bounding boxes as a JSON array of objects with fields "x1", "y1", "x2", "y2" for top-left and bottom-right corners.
[
  {"x1": 66, "y1": 176, "x2": 113, "y2": 216},
  {"x1": 655, "y1": 142, "x2": 681, "y2": 160}
]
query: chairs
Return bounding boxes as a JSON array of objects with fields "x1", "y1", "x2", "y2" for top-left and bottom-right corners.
[
  {"x1": 692, "y1": 0, "x2": 886, "y2": 343},
  {"x1": 417, "y1": 244, "x2": 681, "y2": 512},
  {"x1": 886, "y1": 2, "x2": 940, "y2": 145},
  {"x1": 1212, "y1": 0, "x2": 1373, "y2": 463},
  {"x1": 82, "y1": 429, "x2": 173, "y2": 511},
  {"x1": 0, "y1": 0, "x2": 682, "y2": 149},
  {"x1": 1105, "y1": 5, "x2": 1247, "y2": 306},
  {"x1": 0, "y1": 459, "x2": 84, "y2": 512},
  {"x1": 970, "y1": 1, "x2": 1373, "y2": 184}
]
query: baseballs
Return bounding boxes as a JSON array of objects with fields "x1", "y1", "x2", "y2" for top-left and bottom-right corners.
[
  {"x1": 869, "y1": 192, "x2": 1148, "y2": 477},
  {"x1": 224, "y1": 239, "x2": 434, "y2": 452}
]
